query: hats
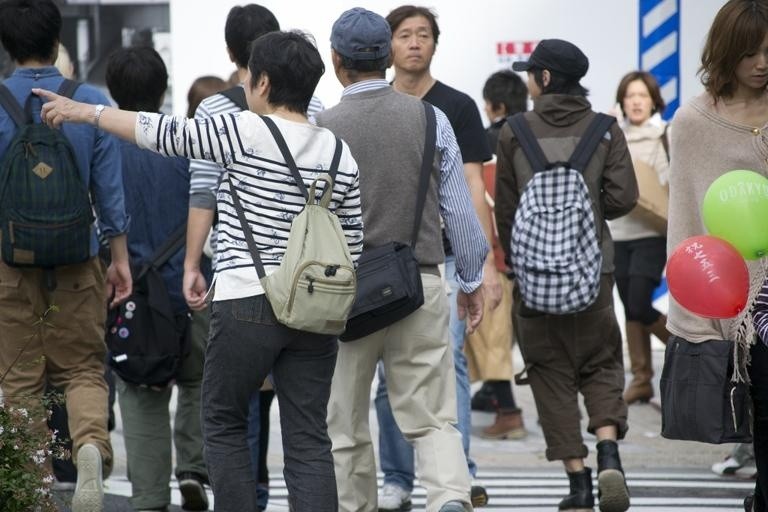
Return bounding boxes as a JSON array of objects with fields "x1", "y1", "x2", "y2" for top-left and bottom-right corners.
[
  {"x1": 512, "y1": 39, "x2": 589, "y2": 80},
  {"x1": 331, "y1": 7, "x2": 392, "y2": 60}
]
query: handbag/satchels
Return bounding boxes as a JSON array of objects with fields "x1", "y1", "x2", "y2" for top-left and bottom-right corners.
[
  {"x1": 658, "y1": 336, "x2": 753, "y2": 440},
  {"x1": 105, "y1": 262, "x2": 189, "y2": 393},
  {"x1": 629, "y1": 159, "x2": 669, "y2": 234},
  {"x1": 338, "y1": 241, "x2": 423, "y2": 342}
]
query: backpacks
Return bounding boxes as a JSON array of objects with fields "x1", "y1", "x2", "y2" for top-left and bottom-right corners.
[
  {"x1": 506, "y1": 112, "x2": 616, "y2": 315},
  {"x1": 229, "y1": 115, "x2": 358, "y2": 337},
  {"x1": 0, "y1": 78, "x2": 95, "y2": 268}
]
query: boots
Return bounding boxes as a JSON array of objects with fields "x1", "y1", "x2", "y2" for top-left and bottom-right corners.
[{"x1": 623, "y1": 315, "x2": 672, "y2": 404}]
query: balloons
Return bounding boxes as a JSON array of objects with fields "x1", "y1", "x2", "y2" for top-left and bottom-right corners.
[
  {"x1": 668, "y1": 237, "x2": 750, "y2": 319},
  {"x1": 707, "y1": 167, "x2": 768, "y2": 263}
]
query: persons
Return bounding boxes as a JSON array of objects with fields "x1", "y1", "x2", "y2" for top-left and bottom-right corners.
[
  {"x1": 0, "y1": 1, "x2": 504, "y2": 511},
  {"x1": 460, "y1": 37, "x2": 673, "y2": 511},
  {"x1": 710, "y1": 442, "x2": 758, "y2": 482},
  {"x1": 662, "y1": 1, "x2": 767, "y2": 512}
]
